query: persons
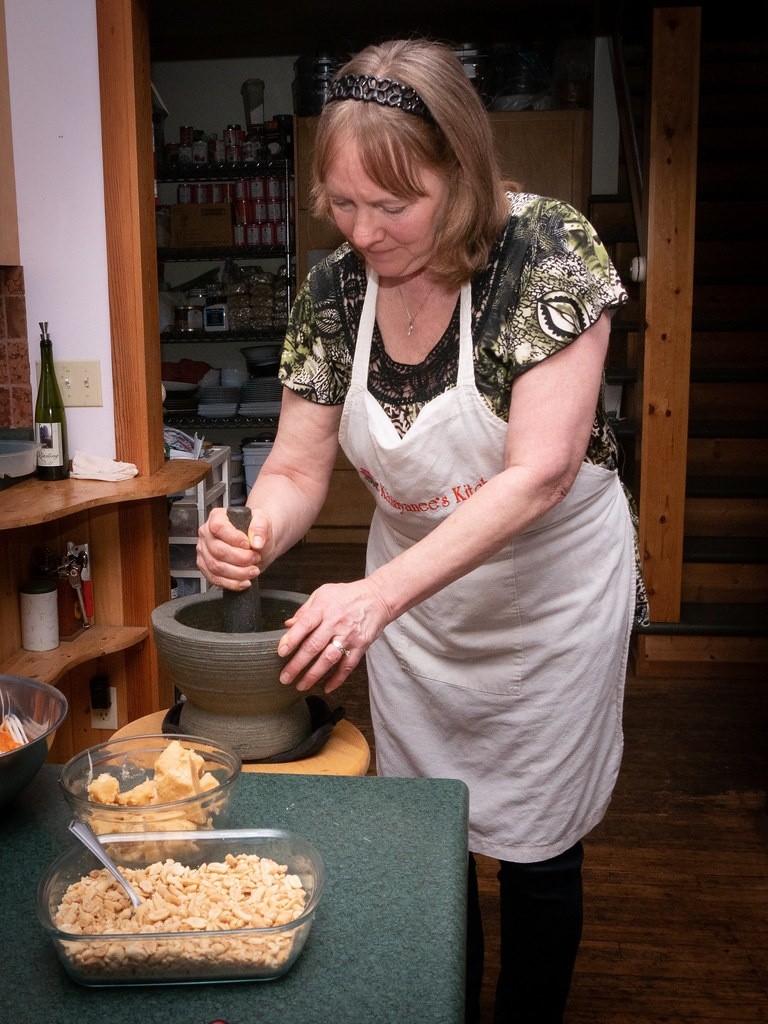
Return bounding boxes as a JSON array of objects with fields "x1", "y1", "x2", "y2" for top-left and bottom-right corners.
[{"x1": 196, "y1": 40, "x2": 651, "y2": 1024}]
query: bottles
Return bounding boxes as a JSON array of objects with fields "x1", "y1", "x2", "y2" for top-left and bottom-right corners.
[
  {"x1": 35, "y1": 321, "x2": 69, "y2": 481},
  {"x1": 174, "y1": 281, "x2": 229, "y2": 335},
  {"x1": 161, "y1": 80, "x2": 295, "y2": 247},
  {"x1": 20, "y1": 582, "x2": 60, "y2": 653}
]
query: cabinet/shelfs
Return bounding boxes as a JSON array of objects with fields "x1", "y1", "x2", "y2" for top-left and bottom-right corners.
[
  {"x1": 155, "y1": 131, "x2": 302, "y2": 428},
  {"x1": 0, "y1": 457, "x2": 211, "y2": 770},
  {"x1": 168, "y1": 445, "x2": 232, "y2": 593},
  {"x1": 285, "y1": 107, "x2": 607, "y2": 546}
]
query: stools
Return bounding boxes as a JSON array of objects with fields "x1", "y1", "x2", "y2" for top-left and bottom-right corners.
[{"x1": 100, "y1": 702, "x2": 373, "y2": 776}]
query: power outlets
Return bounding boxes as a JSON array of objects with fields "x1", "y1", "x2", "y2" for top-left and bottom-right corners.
[
  {"x1": 33, "y1": 361, "x2": 102, "y2": 408},
  {"x1": 90, "y1": 687, "x2": 118, "y2": 730}
]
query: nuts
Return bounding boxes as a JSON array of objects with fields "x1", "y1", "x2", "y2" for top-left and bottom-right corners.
[{"x1": 54, "y1": 852, "x2": 307, "y2": 980}]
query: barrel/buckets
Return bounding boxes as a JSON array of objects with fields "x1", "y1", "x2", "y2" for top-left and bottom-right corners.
[{"x1": 243, "y1": 443, "x2": 274, "y2": 501}]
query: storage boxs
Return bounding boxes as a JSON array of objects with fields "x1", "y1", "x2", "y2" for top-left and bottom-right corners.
[{"x1": 170, "y1": 203, "x2": 233, "y2": 248}]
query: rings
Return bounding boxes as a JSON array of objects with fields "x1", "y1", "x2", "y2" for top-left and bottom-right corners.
[{"x1": 329, "y1": 640, "x2": 350, "y2": 656}]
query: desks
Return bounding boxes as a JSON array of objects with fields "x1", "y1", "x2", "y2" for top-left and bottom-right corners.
[{"x1": 0, "y1": 777, "x2": 483, "y2": 1023}]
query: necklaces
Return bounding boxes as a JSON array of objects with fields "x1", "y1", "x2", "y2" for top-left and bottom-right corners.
[{"x1": 397, "y1": 280, "x2": 438, "y2": 336}]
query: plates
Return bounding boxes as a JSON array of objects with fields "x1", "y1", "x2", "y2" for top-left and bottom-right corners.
[{"x1": 161, "y1": 376, "x2": 283, "y2": 418}]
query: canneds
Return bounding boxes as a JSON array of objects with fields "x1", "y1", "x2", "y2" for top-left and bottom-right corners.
[
  {"x1": 179, "y1": 123, "x2": 256, "y2": 163},
  {"x1": 178, "y1": 176, "x2": 296, "y2": 247}
]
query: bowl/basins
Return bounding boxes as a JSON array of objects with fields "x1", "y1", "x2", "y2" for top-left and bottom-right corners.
[
  {"x1": 150, "y1": 589, "x2": 313, "y2": 760},
  {"x1": 199, "y1": 342, "x2": 282, "y2": 386},
  {"x1": 0, "y1": 674, "x2": 68, "y2": 807},
  {"x1": 58, "y1": 733, "x2": 242, "y2": 864},
  {"x1": 0, "y1": 439, "x2": 42, "y2": 478},
  {"x1": 229, "y1": 456, "x2": 245, "y2": 507},
  {"x1": 35, "y1": 831, "x2": 328, "y2": 985}
]
